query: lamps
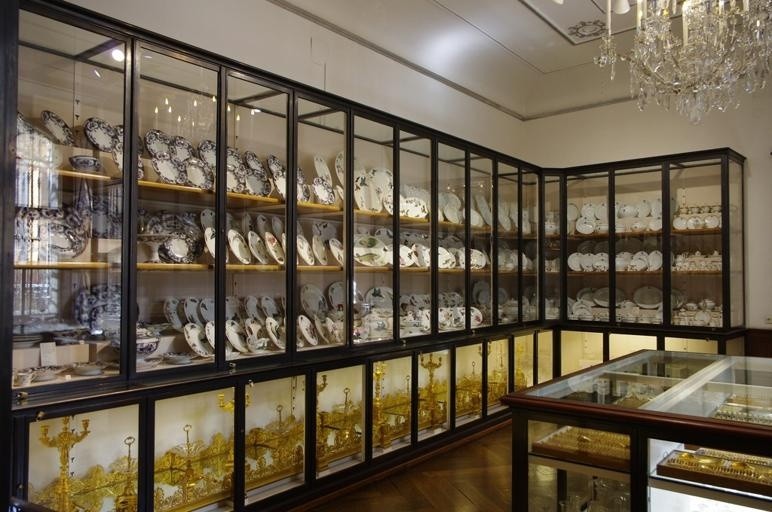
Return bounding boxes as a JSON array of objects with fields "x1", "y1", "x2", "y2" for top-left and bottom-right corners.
[{"x1": 594, "y1": 1, "x2": 772, "y2": 126}]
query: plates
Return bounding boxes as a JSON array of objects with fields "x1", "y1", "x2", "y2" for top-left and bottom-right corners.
[{"x1": 12, "y1": 108, "x2": 739, "y2": 387}]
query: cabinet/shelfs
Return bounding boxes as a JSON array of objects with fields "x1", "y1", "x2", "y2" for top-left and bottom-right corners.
[
  {"x1": 3, "y1": 321, "x2": 560, "y2": 512},
  {"x1": 560, "y1": 145, "x2": 746, "y2": 378},
  {"x1": 2, "y1": 2, "x2": 562, "y2": 405},
  {"x1": 499, "y1": 348, "x2": 772, "y2": 512}
]
